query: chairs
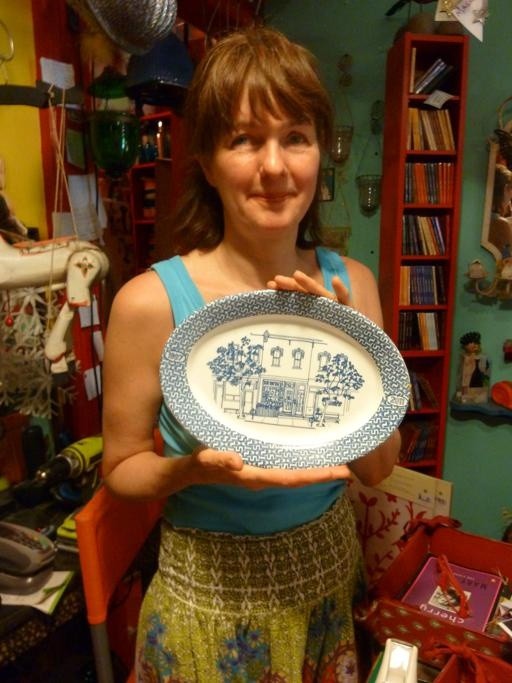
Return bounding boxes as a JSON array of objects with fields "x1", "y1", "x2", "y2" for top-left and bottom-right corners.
[{"x1": 73, "y1": 428, "x2": 169, "y2": 683}]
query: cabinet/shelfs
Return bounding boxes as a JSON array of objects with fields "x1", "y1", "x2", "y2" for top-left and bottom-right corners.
[
  {"x1": 377, "y1": 30, "x2": 470, "y2": 483},
  {"x1": 126, "y1": 112, "x2": 182, "y2": 275}
]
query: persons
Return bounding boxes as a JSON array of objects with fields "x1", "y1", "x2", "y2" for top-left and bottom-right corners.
[
  {"x1": 448, "y1": 329, "x2": 491, "y2": 410},
  {"x1": 96, "y1": 23, "x2": 406, "y2": 682}
]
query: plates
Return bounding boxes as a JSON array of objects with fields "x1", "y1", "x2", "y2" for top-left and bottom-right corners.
[{"x1": 156, "y1": 288, "x2": 414, "y2": 474}]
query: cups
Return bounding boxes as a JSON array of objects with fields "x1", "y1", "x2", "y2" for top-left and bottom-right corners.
[
  {"x1": 354, "y1": 173, "x2": 382, "y2": 215},
  {"x1": 327, "y1": 123, "x2": 354, "y2": 163}
]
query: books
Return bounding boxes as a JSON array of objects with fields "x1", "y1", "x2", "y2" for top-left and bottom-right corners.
[
  {"x1": 392, "y1": 39, "x2": 463, "y2": 476},
  {"x1": 398, "y1": 553, "x2": 506, "y2": 640}
]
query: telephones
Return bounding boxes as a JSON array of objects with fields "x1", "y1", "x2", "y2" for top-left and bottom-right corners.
[{"x1": 0, "y1": 522, "x2": 57, "y2": 575}]
